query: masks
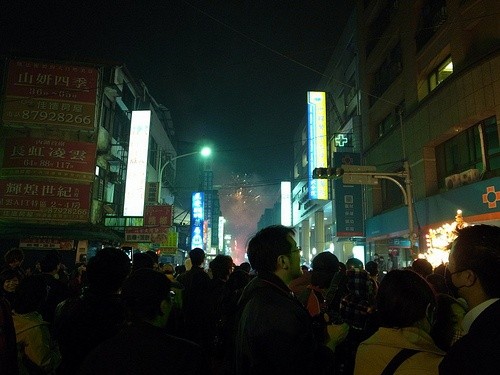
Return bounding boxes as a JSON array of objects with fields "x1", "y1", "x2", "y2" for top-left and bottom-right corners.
[{"x1": 445, "y1": 267, "x2": 472, "y2": 298}]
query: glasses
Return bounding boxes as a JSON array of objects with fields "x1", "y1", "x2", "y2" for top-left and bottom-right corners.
[{"x1": 285, "y1": 246, "x2": 302, "y2": 255}]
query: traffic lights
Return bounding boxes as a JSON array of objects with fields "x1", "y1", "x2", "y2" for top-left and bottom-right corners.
[{"x1": 311, "y1": 167, "x2": 345, "y2": 179}]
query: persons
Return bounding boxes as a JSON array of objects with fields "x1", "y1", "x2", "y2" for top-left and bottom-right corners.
[{"x1": 0, "y1": 221, "x2": 499, "y2": 375}]
